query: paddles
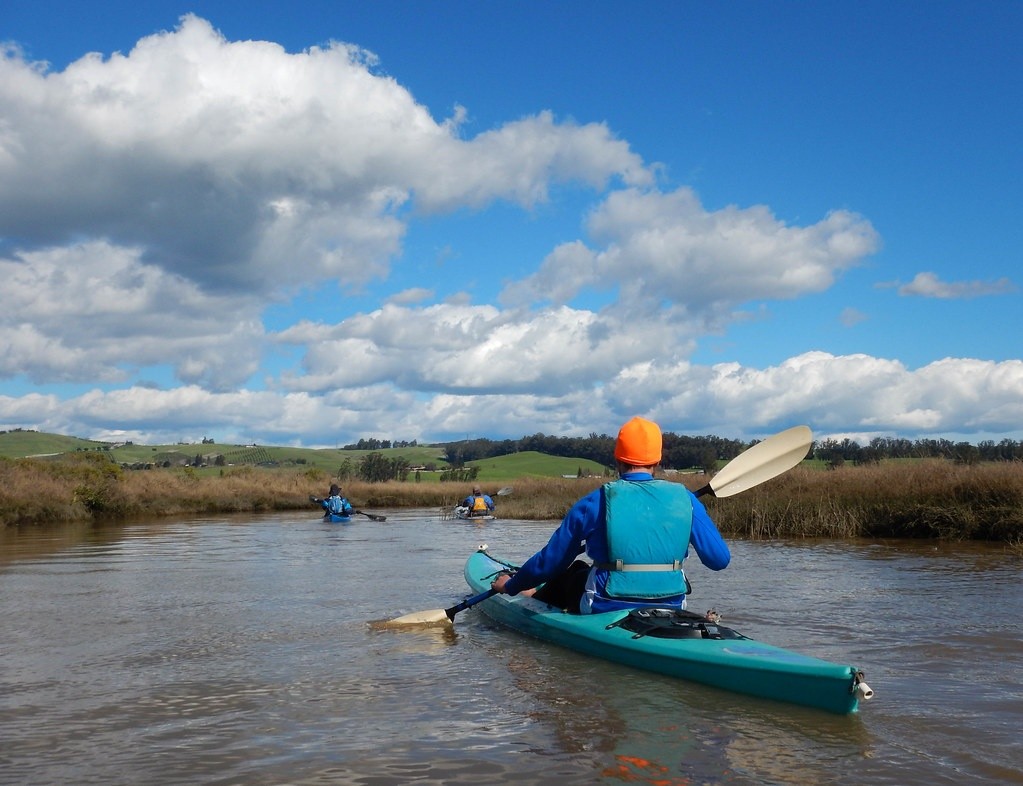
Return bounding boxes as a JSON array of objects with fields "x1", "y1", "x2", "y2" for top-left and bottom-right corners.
[
  {"x1": 489, "y1": 486, "x2": 514, "y2": 498},
  {"x1": 385, "y1": 424, "x2": 815, "y2": 624},
  {"x1": 308, "y1": 494, "x2": 387, "y2": 523}
]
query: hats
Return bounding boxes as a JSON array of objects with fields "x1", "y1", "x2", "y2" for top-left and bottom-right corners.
[
  {"x1": 329, "y1": 484, "x2": 342, "y2": 495},
  {"x1": 614, "y1": 417, "x2": 662, "y2": 466}
]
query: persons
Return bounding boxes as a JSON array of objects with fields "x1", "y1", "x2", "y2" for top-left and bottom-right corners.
[
  {"x1": 491, "y1": 417, "x2": 730, "y2": 615},
  {"x1": 322, "y1": 483, "x2": 361, "y2": 518},
  {"x1": 462, "y1": 487, "x2": 495, "y2": 517}
]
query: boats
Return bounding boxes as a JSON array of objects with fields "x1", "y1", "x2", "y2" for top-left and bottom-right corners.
[
  {"x1": 325, "y1": 512, "x2": 350, "y2": 524},
  {"x1": 455, "y1": 505, "x2": 496, "y2": 521},
  {"x1": 461, "y1": 543, "x2": 874, "y2": 716}
]
